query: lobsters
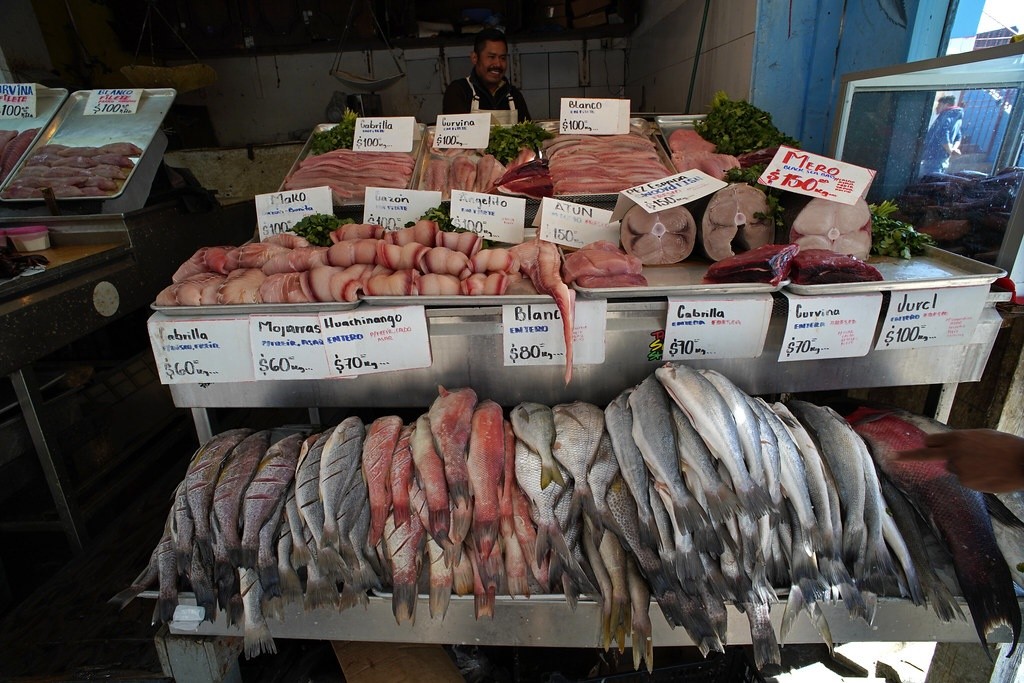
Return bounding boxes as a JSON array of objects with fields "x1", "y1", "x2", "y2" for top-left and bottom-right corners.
[{"x1": 0, "y1": 235, "x2": 50, "y2": 280}]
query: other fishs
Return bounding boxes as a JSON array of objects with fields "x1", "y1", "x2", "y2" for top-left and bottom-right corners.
[
  {"x1": 0, "y1": 127, "x2": 143, "y2": 199},
  {"x1": 156, "y1": 118, "x2": 887, "y2": 285},
  {"x1": 103, "y1": 361, "x2": 1023, "y2": 674}
]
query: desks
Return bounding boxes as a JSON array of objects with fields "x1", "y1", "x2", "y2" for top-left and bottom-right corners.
[{"x1": 0, "y1": 244, "x2": 146, "y2": 565}]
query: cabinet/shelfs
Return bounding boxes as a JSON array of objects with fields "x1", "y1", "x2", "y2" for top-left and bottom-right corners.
[{"x1": 826, "y1": 39, "x2": 1024, "y2": 285}]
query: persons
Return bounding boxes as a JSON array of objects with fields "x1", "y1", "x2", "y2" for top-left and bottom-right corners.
[
  {"x1": 440, "y1": 27, "x2": 536, "y2": 126},
  {"x1": 918, "y1": 95, "x2": 966, "y2": 175}
]
